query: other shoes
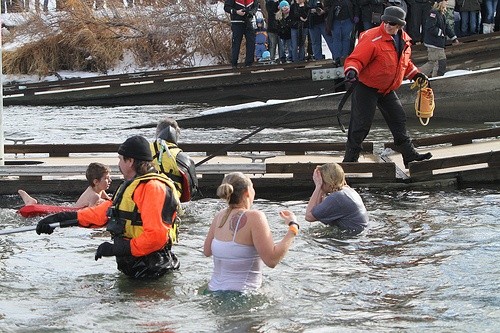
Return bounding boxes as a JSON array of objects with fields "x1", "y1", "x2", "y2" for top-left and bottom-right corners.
[{"x1": 404, "y1": 152, "x2": 433, "y2": 169}]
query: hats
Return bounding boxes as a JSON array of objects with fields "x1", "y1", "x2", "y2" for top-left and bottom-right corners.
[
  {"x1": 278, "y1": 0, "x2": 290, "y2": 9},
  {"x1": 380, "y1": 6, "x2": 407, "y2": 27},
  {"x1": 118, "y1": 135, "x2": 154, "y2": 162}
]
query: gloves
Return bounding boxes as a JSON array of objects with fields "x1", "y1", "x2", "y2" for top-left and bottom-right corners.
[
  {"x1": 35, "y1": 210, "x2": 78, "y2": 236},
  {"x1": 345, "y1": 69, "x2": 357, "y2": 84},
  {"x1": 413, "y1": 72, "x2": 431, "y2": 88},
  {"x1": 94, "y1": 241, "x2": 132, "y2": 261}
]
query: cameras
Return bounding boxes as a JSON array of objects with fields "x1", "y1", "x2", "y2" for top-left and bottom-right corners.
[{"x1": 242, "y1": 9, "x2": 253, "y2": 18}]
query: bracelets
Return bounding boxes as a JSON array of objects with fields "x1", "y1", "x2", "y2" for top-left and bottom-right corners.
[
  {"x1": 289, "y1": 221, "x2": 299, "y2": 229},
  {"x1": 289, "y1": 226, "x2": 299, "y2": 235}
]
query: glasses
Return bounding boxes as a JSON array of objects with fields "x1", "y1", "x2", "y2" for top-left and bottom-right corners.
[{"x1": 383, "y1": 20, "x2": 398, "y2": 26}]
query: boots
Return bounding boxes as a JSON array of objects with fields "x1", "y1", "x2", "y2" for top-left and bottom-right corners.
[{"x1": 483, "y1": 23, "x2": 495, "y2": 35}]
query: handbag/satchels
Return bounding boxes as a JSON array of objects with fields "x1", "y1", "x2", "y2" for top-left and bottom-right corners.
[{"x1": 371, "y1": 11, "x2": 382, "y2": 24}]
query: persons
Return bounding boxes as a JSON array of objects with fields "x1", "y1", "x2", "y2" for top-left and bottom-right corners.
[
  {"x1": 444, "y1": 0, "x2": 500, "y2": 41},
  {"x1": 224, "y1": 0, "x2": 258, "y2": 68},
  {"x1": 36, "y1": 136, "x2": 183, "y2": 278},
  {"x1": 304, "y1": 162, "x2": 368, "y2": 229},
  {"x1": 203, "y1": 172, "x2": 299, "y2": 292},
  {"x1": 254, "y1": 18, "x2": 269, "y2": 62},
  {"x1": 265, "y1": 0, "x2": 434, "y2": 68},
  {"x1": 342, "y1": 6, "x2": 433, "y2": 169},
  {"x1": 18, "y1": 162, "x2": 112, "y2": 209},
  {"x1": 418, "y1": 0, "x2": 459, "y2": 78},
  {"x1": 150, "y1": 118, "x2": 198, "y2": 203}
]
